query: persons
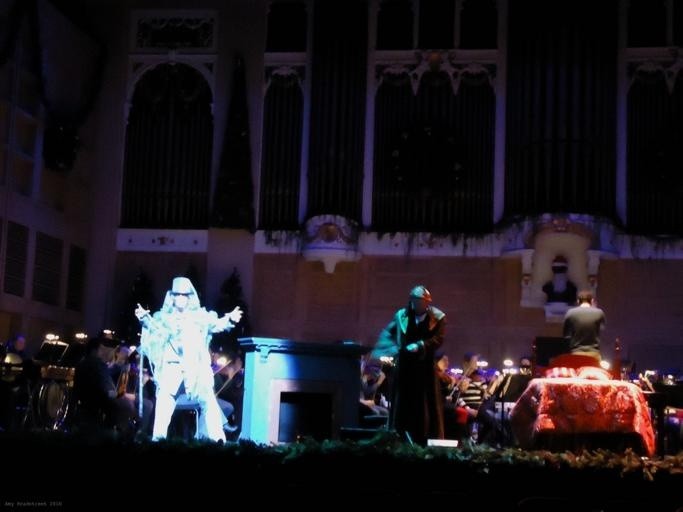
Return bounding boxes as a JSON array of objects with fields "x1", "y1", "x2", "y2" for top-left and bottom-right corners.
[
  {"x1": 561, "y1": 291, "x2": 604, "y2": 364},
  {"x1": 175, "y1": 346, "x2": 238, "y2": 434},
  {"x1": 358, "y1": 352, "x2": 532, "y2": 446},
  {"x1": 370, "y1": 286, "x2": 448, "y2": 450},
  {"x1": 107, "y1": 344, "x2": 153, "y2": 441},
  {"x1": 7, "y1": 334, "x2": 26, "y2": 361},
  {"x1": 133, "y1": 276, "x2": 243, "y2": 442}
]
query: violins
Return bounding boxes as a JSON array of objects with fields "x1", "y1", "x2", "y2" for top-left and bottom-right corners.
[
  {"x1": 116, "y1": 347, "x2": 138, "y2": 399},
  {"x1": 443, "y1": 369, "x2": 500, "y2": 390}
]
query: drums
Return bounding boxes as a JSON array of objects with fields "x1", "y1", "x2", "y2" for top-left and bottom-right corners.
[
  {"x1": 30, "y1": 382, "x2": 67, "y2": 427},
  {"x1": 0, "y1": 375, "x2": 19, "y2": 391},
  {"x1": 40, "y1": 365, "x2": 75, "y2": 383}
]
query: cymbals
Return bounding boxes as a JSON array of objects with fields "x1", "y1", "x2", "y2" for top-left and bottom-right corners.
[
  {"x1": 44, "y1": 340, "x2": 67, "y2": 346},
  {"x1": 2, "y1": 352, "x2": 22, "y2": 364}
]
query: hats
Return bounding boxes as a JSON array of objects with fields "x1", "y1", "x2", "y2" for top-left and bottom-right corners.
[{"x1": 171, "y1": 277, "x2": 194, "y2": 296}]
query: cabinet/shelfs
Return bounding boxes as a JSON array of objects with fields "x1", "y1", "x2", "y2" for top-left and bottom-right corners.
[{"x1": 236, "y1": 336, "x2": 368, "y2": 446}]
